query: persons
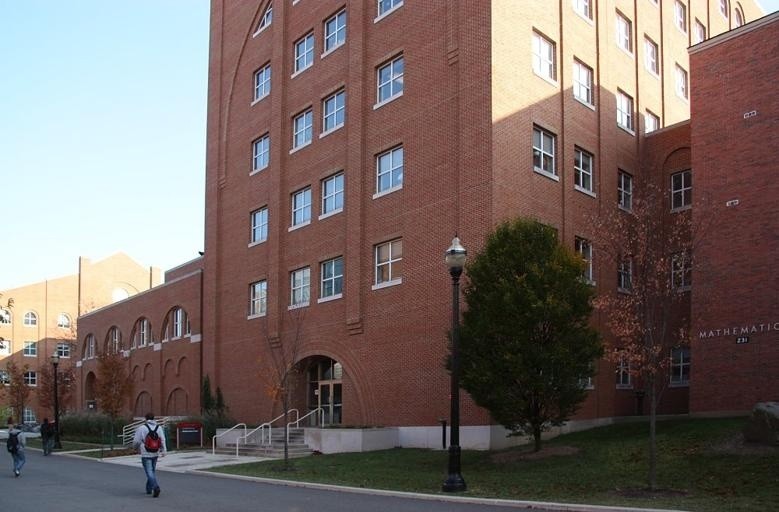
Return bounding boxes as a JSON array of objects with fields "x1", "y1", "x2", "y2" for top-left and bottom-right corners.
[
  {"x1": 7, "y1": 424, "x2": 26, "y2": 477},
  {"x1": 132, "y1": 413, "x2": 167, "y2": 498},
  {"x1": 40, "y1": 418, "x2": 52, "y2": 456}
]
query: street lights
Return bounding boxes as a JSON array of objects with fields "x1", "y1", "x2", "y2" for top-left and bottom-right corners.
[
  {"x1": 50, "y1": 351, "x2": 63, "y2": 449},
  {"x1": 446, "y1": 233, "x2": 467, "y2": 491}
]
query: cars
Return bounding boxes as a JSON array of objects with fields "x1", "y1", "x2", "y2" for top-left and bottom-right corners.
[{"x1": 0, "y1": 419, "x2": 58, "y2": 442}]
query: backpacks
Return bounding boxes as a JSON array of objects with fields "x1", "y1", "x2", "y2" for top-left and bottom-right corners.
[
  {"x1": 7, "y1": 433, "x2": 19, "y2": 453},
  {"x1": 145, "y1": 432, "x2": 160, "y2": 452}
]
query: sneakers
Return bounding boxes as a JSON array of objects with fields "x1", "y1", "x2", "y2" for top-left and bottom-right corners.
[
  {"x1": 146, "y1": 489, "x2": 152, "y2": 494},
  {"x1": 153, "y1": 487, "x2": 160, "y2": 497}
]
